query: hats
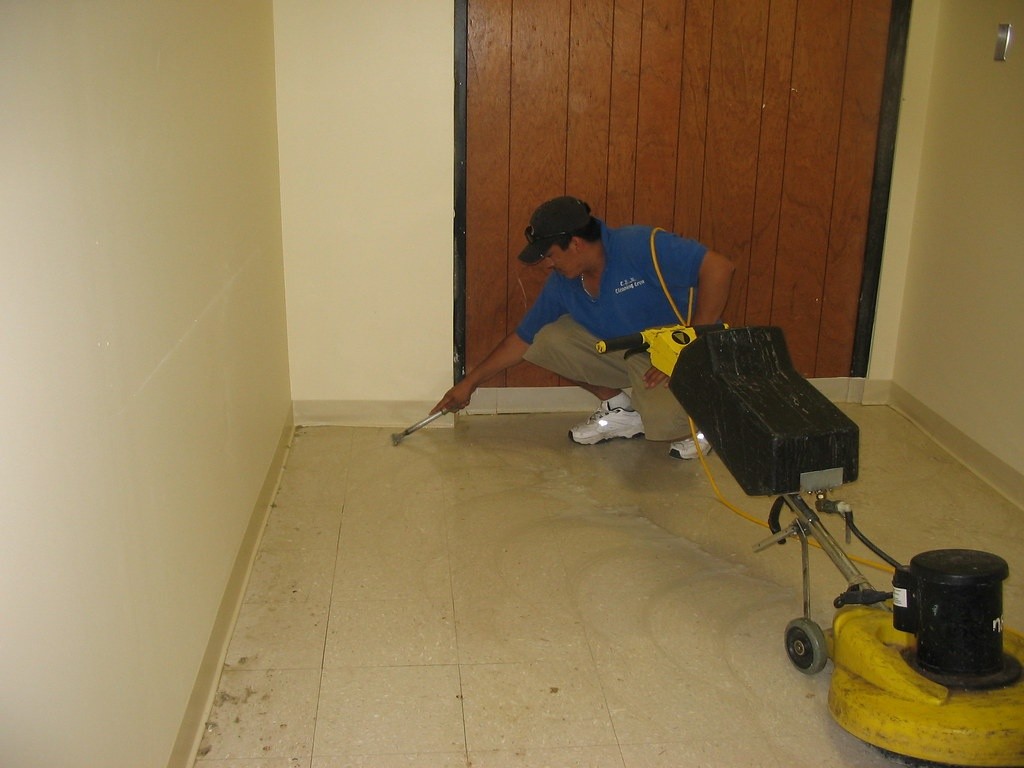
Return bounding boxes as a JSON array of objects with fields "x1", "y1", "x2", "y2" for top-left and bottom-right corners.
[{"x1": 518, "y1": 195, "x2": 592, "y2": 264}]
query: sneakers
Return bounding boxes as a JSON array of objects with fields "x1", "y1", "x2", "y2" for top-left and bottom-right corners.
[
  {"x1": 569, "y1": 400, "x2": 645, "y2": 445},
  {"x1": 669, "y1": 431, "x2": 712, "y2": 461}
]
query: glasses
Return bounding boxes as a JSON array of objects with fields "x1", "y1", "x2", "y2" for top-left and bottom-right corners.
[{"x1": 524, "y1": 225, "x2": 568, "y2": 246}]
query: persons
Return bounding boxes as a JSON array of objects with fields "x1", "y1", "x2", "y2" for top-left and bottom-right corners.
[{"x1": 428, "y1": 196, "x2": 735, "y2": 460}]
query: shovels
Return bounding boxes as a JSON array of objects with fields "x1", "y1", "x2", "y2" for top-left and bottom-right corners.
[{"x1": 391, "y1": 406, "x2": 449, "y2": 446}]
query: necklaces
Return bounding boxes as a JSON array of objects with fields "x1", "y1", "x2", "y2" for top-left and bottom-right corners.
[{"x1": 580, "y1": 272, "x2": 591, "y2": 297}]
